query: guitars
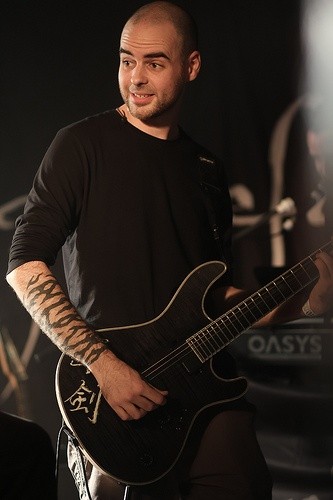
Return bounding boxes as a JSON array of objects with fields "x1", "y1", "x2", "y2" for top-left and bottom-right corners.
[{"x1": 56, "y1": 238, "x2": 333, "y2": 488}]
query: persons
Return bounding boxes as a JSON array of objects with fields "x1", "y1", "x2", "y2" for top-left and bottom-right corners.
[{"x1": 6, "y1": 3, "x2": 333, "y2": 500}]
